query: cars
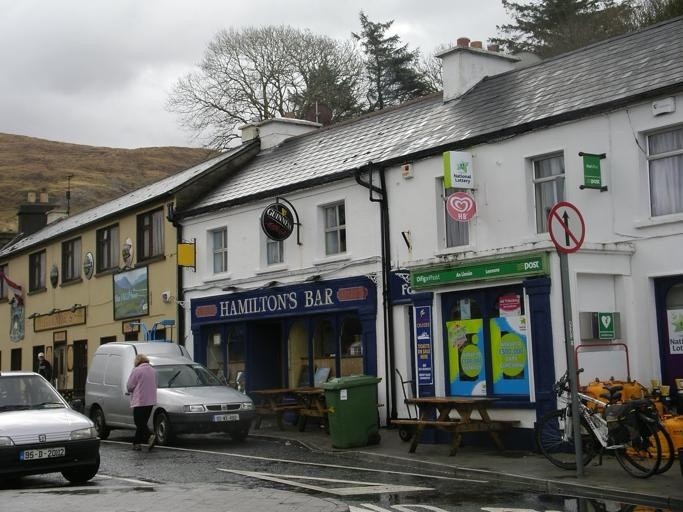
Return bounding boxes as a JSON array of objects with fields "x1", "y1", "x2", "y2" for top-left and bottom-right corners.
[{"x1": 0, "y1": 371, "x2": 101, "y2": 482}]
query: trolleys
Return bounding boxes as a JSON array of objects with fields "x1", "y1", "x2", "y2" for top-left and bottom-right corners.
[{"x1": 395, "y1": 368, "x2": 417, "y2": 442}]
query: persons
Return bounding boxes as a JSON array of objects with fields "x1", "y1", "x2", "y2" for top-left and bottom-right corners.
[
  {"x1": 125, "y1": 353, "x2": 160, "y2": 452},
  {"x1": 36, "y1": 352, "x2": 51, "y2": 382}
]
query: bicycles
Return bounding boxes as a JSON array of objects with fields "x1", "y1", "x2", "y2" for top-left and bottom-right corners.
[{"x1": 536, "y1": 368, "x2": 674, "y2": 479}]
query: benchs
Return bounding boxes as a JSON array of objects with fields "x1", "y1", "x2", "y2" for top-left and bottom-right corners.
[
  {"x1": 391, "y1": 394, "x2": 521, "y2": 459},
  {"x1": 247, "y1": 387, "x2": 328, "y2": 435}
]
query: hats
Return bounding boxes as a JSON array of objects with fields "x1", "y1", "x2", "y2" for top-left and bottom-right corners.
[{"x1": 38, "y1": 352, "x2": 45, "y2": 357}]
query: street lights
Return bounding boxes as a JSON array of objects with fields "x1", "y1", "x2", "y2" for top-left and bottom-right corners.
[{"x1": 128, "y1": 320, "x2": 175, "y2": 341}]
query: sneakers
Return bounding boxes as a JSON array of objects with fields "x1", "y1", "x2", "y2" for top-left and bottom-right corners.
[{"x1": 132, "y1": 432, "x2": 157, "y2": 453}]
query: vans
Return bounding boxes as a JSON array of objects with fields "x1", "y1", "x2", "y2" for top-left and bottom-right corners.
[{"x1": 84, "y1": 341, "x2": 256, "y2": 483}]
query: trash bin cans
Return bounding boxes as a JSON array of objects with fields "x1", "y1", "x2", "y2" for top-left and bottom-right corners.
[{"x1": 319, "y1": 374, "x2": 382, "y2": 448}]
